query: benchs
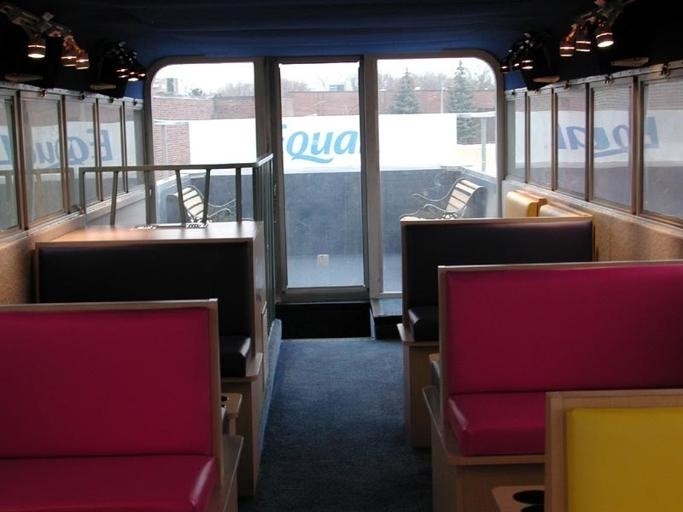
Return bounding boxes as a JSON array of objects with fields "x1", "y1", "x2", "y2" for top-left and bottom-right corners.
[
  {"x1": 399, "y1": 188, "x2": 683, "y2": 512},
  {"x1": 1, "y1": 202, "x2": 269, "y2": 512}
]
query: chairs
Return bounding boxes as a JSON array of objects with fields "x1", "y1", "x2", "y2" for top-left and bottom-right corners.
[
  {"x1": 398, "y1": 177, "x2": 486, "y2": 221},
  {"x1": 168, "y1": 184, "x2": 237, "y2": 223}
]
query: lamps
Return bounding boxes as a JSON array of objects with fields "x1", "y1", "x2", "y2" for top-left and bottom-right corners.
[
  {"x1": 109, "y1": 41, "x2": 146, "y2": 85},
  {"x1": 554, "y1": 0, "x2": 632, "y2": 58},
  {"x1": 49, "y1": 28, "x2": 90, "y2": 69},
  {"x1": 19, "y1": 23, "x2": 47, "y2": 60},
  {"x1": 498, "y1": 29, "x2": 553, "y2": 72}
]
